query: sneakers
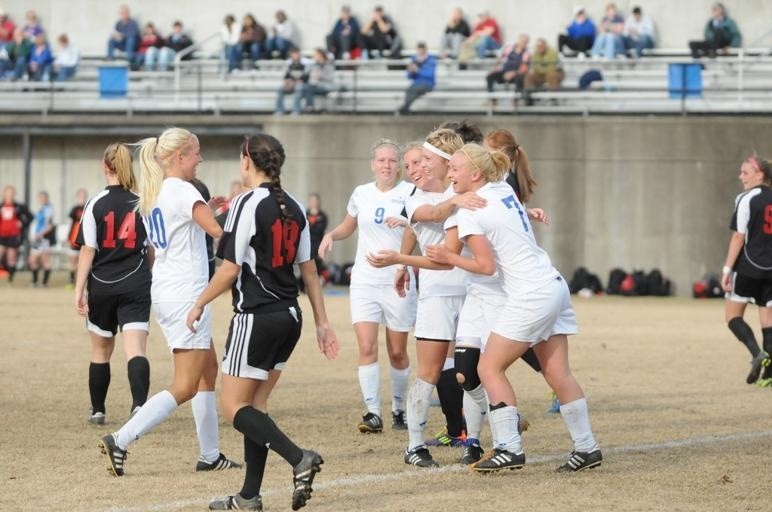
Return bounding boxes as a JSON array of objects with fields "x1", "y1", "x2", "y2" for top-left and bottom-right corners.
[
  {"x1": 555, "y1": 448, "x2": 603, "y2": 474},
  {"x1": 470, "y1": 448, "x2": 526, "y2": 473},
  {"x1": 98, "y1": 433, "x2": 129, "y2": 479},
  {"x1": 547, "y1": 391, "x2": 561, "y2": 413},
  {"x1": 424, "y1": 426, "x2": 466, "y2": 448},
  {"x1": 291, "y1": 449, "x2": 324, "y2": 512},
  {"x1": 195, "y1": 451, "x2": 243, "y2": 471},
  {"x1": 357, "y1": 411, "x2": 383, "y2": 434},
  {"x1": 517, "y1": 418, "x2": 529, "y2": 435},
  {"x1": 460, "y1": 445, "x2": 485, "y2": 468},
  {"x1": 391, "y1": 409, "x2": 408, "y2": 431},
  {"x1": 402, "y1": 443, "x2": 440, "y2": 468},
  {"x1": 208, "y1": 492, "x2": 263, "y2": 512},
  {"x1": 86, "y1": 412, "x2": 106, "y2": 425},
  {"x1": 747, "y1": 349, "x2": 772, "y2": 389},
  {"x1": 130, "y1": 405, "x2": 142, "y2": 419}
]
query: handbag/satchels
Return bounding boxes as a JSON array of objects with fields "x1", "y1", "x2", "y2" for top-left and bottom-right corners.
[
  {"x1": 567, "y1": 265, "x2": 604, "y2": 295},
  {"x1": 692, "y1": 276, "x2": 726, "y2": 298},
  {"x1": 604, "y1": 268, "x2": 672, "y2": 298}
]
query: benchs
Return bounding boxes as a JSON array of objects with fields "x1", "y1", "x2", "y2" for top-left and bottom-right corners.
[{"x1": 0, "y1": 50, "x2": 771, "y2": 119}]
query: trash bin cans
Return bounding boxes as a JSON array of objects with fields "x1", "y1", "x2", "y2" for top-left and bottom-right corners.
[
  {"x1": 97, "y1": 65, "x2": 127, "y2": 98},
  {"x1": 668, "y1": 62, "x2": 706, "y2": 99}
]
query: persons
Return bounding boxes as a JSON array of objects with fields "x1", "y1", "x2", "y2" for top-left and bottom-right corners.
[
  {"x1": 305, "y1": 193, "x2": 334, "y2": 287},
  {"x1": 187, "y1": 177, "x2": 251, "y2": 284},
  {"x1": 98, "y1": 127, "x2": 243, "y2": 478},
  {"x1": 26, "y1": 189, "x2": 57, "y2": 286},
  {"x1": 65, "y1": 188, "x2": 90, "y2": 283},
  {"x1": 71, "y1": 142, "x2": 157, "y2": 426},
  {"x1": 186, "y1": 133, "x2": 343, "y2": 511},
  {"x1": 1, "y1": 184, "x2": 34, "y2": 282}
]
